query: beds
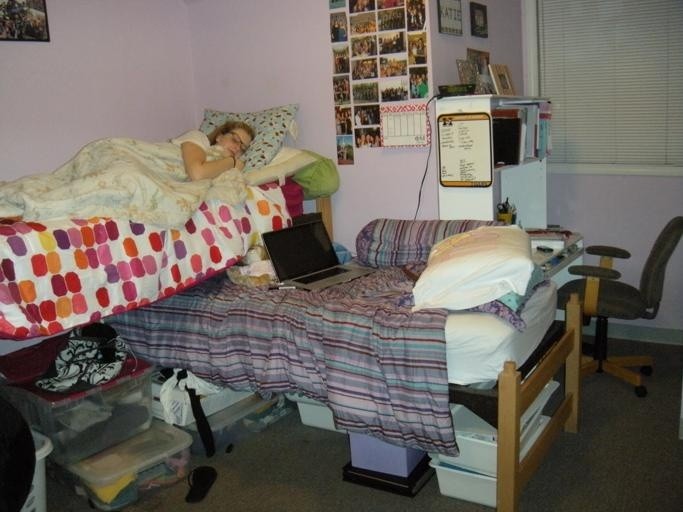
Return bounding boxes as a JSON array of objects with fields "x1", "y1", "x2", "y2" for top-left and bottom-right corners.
[
  {"x1": 1, "y1": 129, "x2": 333, "y2": 353},
  {"x1": 115, "y1": 259, "x2": 584, "y2": 512}
]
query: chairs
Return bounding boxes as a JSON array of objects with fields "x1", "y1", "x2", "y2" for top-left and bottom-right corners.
[{"x1": 557, "y1": 217, "x2": 681, "y2": 402}]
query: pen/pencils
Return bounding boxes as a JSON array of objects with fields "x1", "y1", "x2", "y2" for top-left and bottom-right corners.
[{"x1": 268, "y1": 285, "x2": 296, "y2": 291}]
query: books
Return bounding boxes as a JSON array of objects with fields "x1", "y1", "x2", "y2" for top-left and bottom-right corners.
[{"x1": 491, "y1": 100, "x2": 554, "y2": 167}]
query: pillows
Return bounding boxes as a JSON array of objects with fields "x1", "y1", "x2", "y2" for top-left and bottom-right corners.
[
  {"x1": 201, "y1": 101, "x2": 341, "y2": 199},
  {"x1": 354, "y1": 218, "x2": 544, "y2": 316}
]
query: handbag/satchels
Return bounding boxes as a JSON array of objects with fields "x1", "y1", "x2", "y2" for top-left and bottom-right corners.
[{"x1": 34, "y1": 322, "x2": 137, "y2": 392}]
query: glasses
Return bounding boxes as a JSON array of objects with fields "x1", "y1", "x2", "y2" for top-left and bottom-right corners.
[{"x1": 230, "y1": 131, "x2": 247, "y2": 152}]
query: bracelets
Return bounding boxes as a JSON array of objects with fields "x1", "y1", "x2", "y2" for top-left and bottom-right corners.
[{"x1": 230, "y1": 156, "x2": 237, "y2": 168}]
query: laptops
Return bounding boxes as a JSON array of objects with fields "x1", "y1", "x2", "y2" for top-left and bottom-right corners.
[{"x1": 261, "y1": 219, "x2": 377, "y2": 293}]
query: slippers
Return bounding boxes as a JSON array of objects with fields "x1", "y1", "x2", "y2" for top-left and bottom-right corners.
[{"x1": 185, "y1": 465, "x2": 218, "y2": 504}]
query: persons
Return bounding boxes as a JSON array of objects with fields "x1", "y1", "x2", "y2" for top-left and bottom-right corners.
[
  {"x1": 499, "y1": 75, "x2": 508, "y2": 89},
  {"x1": 165, "y1": 121, "x2": 255, "y2": 182},
  {"x1": 0, "y1": 0, "x2": 46, "y2": 41},
  {"x1": 330, "y1": 0, "x2": 429, "y2": 163}
]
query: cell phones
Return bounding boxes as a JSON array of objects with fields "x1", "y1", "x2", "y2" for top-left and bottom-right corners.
[{"x1": 537, "y1": 245, "x2": 554, "y2": 252}]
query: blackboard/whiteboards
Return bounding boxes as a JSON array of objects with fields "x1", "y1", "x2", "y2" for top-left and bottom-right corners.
[{"x1": 438, "y1": 113, "x2": 493, "y2": 188}]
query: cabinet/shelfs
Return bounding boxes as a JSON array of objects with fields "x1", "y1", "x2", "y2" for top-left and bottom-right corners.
[{"x1": 438, "y1": 93, "x2": 583, "y2": 316}]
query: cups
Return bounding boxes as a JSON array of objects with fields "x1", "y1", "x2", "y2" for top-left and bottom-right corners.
[{"x1": 496, "y1": 213, "x2": 512, "y2": 226}]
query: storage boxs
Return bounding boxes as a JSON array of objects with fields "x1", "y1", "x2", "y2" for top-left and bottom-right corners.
[
  {"x1": 285, "y1": 382, "x2": 558, "y2": 508},
  {"x1": 14, "y1": 360, "x2": 197, "y2": 509}
]
